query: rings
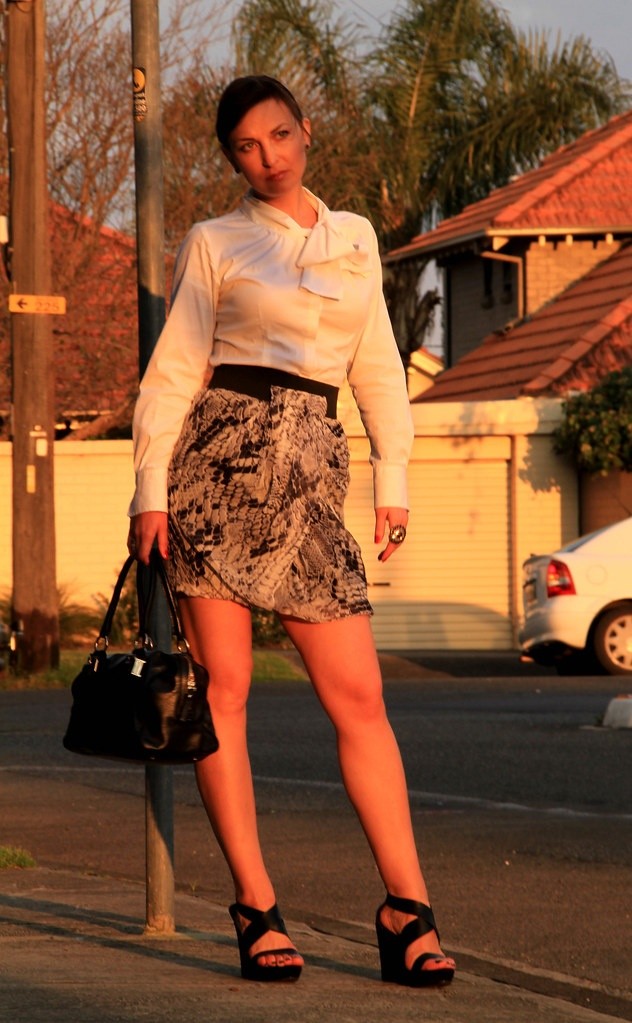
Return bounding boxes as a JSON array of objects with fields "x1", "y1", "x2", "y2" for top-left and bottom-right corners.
[{"x1": 389, "y1": 525, "x2": 406, "y2": 543}]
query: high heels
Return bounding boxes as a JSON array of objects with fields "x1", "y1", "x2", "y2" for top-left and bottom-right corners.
[
  {"x1": 229, "y1": 901, "x2": 304, "y2": 985},
  {"x1": 375, "y1": 888, "x2": 455, "y2": 988}
]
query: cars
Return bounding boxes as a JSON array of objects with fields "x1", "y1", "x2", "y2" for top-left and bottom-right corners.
[{"x1": 518, "y1": 515, "x2": 632, "y2": 676}]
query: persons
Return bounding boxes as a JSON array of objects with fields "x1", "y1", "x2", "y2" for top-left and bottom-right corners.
[{"x1": 128, "y1": 76, "x2": 459, "y2": 989}]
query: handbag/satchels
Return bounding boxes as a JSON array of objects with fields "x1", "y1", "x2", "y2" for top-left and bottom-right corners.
[{"x1": 63, "y1": 551, "x2": 221, "y2": 765}]
